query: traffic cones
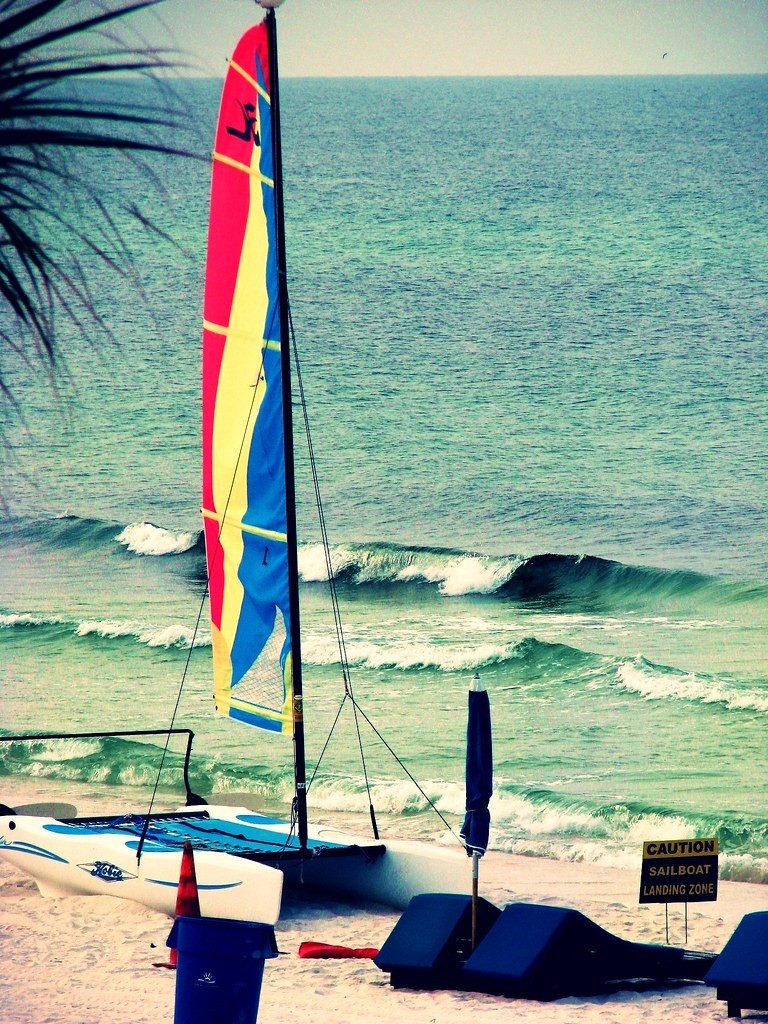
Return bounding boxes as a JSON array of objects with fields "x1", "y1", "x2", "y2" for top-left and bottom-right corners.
[{"x1": 152, "y1": 839, "x2": 201, "y2": 973}]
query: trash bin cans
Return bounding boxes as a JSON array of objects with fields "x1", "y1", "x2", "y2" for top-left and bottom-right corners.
[{"x1": 163, "y1": 916, "x2": 279, "y2": 1024}]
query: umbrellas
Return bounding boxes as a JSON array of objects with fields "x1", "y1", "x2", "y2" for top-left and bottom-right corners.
[{"x1": 461, "y1": 673, "x2": 494, "y2": 950}]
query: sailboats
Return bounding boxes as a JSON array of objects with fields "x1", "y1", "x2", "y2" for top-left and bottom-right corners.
[{"x1": 0, "y1": 0, "x2": 430, "y2": 928}]
